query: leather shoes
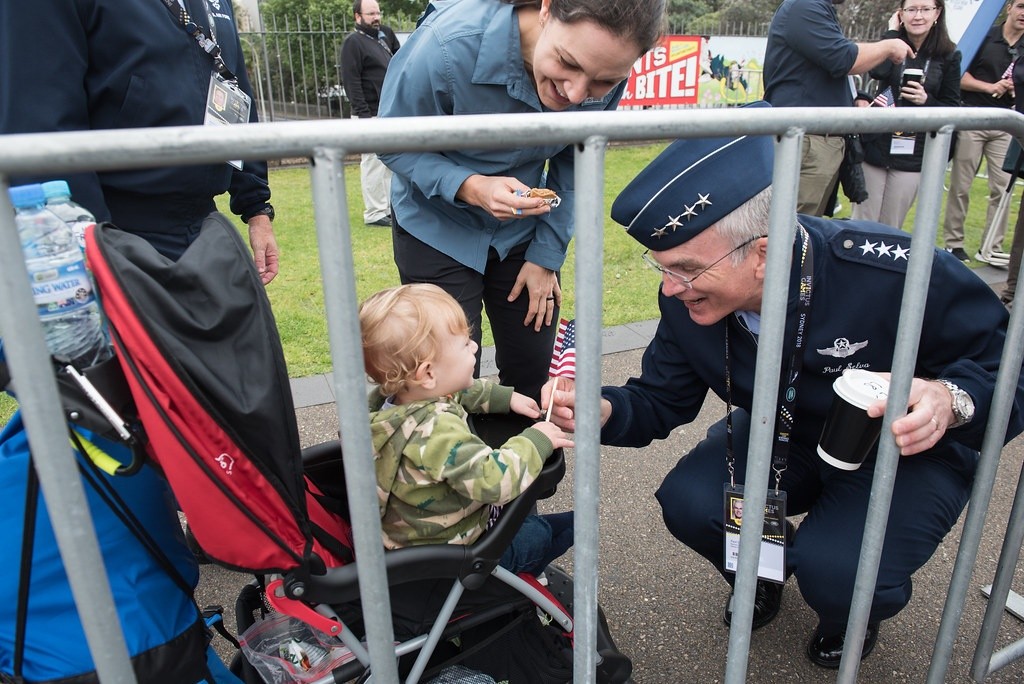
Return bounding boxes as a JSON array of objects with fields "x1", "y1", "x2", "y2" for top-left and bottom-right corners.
[
  {"x1": 723, "y1": 523, "x2": 796, "y2": 629},
  {"x1": 806, "y1": 620, "x2": 882, "y2": 666}
]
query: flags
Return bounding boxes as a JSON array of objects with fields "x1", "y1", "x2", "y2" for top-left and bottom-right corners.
[
  {"x1": 875, "y1": 88, "x2": 895, "y2": 107},
  {"x1": 1001, "y1": 61, "x2": 1016, "y2": 79},
  {"x1": 548, "y1": 318, "x2": 575, "y2": 378}
]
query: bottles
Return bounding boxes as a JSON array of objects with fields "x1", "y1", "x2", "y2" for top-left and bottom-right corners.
[
  {"x1": 901, "y1": 68, "x2": 923, "y2": 98},
  {"x1": 42, "y1": 180, "x2": 97, "y2": 255},
  {"x1": 8, "y1": 183, "x2": 101, "y2": 362}
]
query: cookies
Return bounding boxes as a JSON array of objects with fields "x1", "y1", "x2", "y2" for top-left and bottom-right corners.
[{"x1": 529, "y1": 188, "x2": 558, "y2": 199}]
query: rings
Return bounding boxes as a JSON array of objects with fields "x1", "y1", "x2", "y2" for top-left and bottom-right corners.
[
  {"x1": 932, "y1": 419, "x2": 938, "y2": 430},
  {"x1": 547, "y1": 296, "x2": 554, "y2": 300}
]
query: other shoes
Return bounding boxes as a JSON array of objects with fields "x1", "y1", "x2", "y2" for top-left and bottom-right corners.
[
  {"x1": 944, "y1": 247, "x2": 971, "y2": 263},
  {"x1": 832, "y1": 203, "x2": 841, "y2": 214},
  {"x1": 975, "y1": 249, "x2": 1007, "y2": 266},
  {"x1": 366, "y1": 214, "x2": 391, "y2": 226}
]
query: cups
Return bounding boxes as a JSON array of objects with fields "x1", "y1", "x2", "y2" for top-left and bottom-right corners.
[{"x1": 817, "y1": 368, "x2": 890, "y2": 470}]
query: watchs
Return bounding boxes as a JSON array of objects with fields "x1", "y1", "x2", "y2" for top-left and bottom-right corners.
[{"x1": 935, "y1": 378, "x2": 975, "y2": 427}]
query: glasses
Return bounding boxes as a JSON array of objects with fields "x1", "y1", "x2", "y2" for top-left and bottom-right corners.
[
  {"x1": 641, "y1": 235, "x2": 768, "y2": 290},
  {"x1": 359, "y1": 12, "x2": 381, "y2": 17},
  {"x1": 903, "y1": 6, "x2": 937, "y2": 15}
]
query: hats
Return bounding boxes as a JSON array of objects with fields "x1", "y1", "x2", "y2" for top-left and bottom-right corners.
[{"x1": 611, "y1": 101, "x2": 775, "y2": 252}]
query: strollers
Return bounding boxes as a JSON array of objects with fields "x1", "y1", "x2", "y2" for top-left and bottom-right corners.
[{"x1": 0, "y1": 213, "x2": 634, "y2": 684}]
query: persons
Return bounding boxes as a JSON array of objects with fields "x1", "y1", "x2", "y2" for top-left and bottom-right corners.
[
  {"x1": 537, "y1": 98, "x2": 1024, "y2": 664},
  {"x1": 341, "y1": 0, "x2": 396, "y2": 226},
  {"x1": 846, "y1": 0, "x2": 963, "y2": 234},
  {"x1": 942, "y1": 1, "x2": 1024, "y2": 269},
  {"x1": 358, "y1": 283, "x2": 575, "y2": 580},
  {"x1": 763, "y1": 0, "x2": 916, "y2": 217},
  {"x1": 999, "y1": 53, "x2": 1024, "y2": 304},
  {"x1": 1, "y1": 0, "x2": 280, "y2": 577},
  {"x1": 376, "y1": 0, "x2": 668, "y2": 406}
]
